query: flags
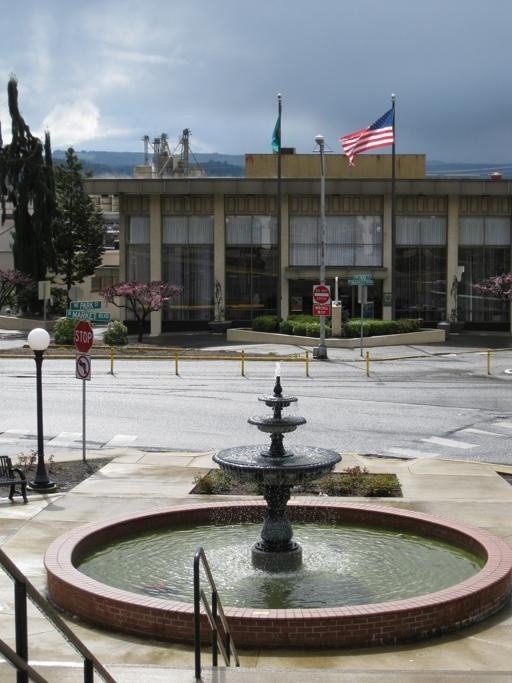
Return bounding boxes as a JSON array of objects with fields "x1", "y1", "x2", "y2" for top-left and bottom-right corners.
[
  {"x1": 269, "y1": 92, "x2": 282, "y2": 160},
  {"x1": 337, "y1": 92, "x2": 396, "y2": 167}
]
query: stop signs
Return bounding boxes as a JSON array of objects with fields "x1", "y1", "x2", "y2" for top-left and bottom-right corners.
[
  {"x1": 312, "y1": 285, "x2": 331, "y2": 305},
  {"x1": 73, "y1": 320, "x2": 93, "y2": 353}
]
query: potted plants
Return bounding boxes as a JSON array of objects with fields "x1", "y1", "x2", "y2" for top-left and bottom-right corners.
[{"x1": 436, "y1": 274, "x2": 465, "y2": 342}]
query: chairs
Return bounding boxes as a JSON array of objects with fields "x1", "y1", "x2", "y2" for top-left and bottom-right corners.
[{"x1": 0, "y1": 456, "x2": 29, "y2": 506}]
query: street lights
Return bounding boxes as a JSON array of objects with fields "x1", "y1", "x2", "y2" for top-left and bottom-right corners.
[
  {"x1": 25, "y1": 328, "x2": 56, "y2": 493},
  {"x1": 314, "y1": 134, "x2": 327, "y2": 359}
]
